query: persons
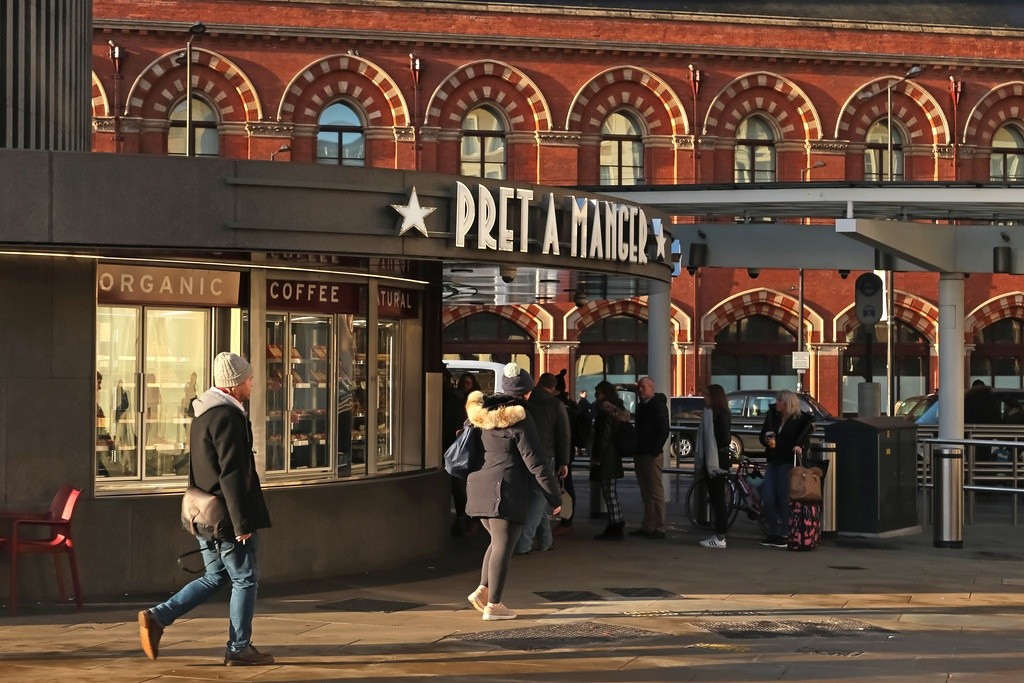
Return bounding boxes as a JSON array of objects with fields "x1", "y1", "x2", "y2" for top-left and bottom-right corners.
[
  {"x1": 759, "y1": 390, "x2": 815, "y2": 550},
  {"x1": 463, "y1": 362, "x2": 563, "y2": 621},
  {"x1": 137, "y1": 351, "x2": 275, "y2": 667},
  {"x1": 95, "y1": 371, "x2": 202, "y2": 477},
  {"x1": 698, "y1": 383, "x2": 732, "y2": 550},
  {"x1": 442, "y1": 361, "x2": 670, "y2": 554}
]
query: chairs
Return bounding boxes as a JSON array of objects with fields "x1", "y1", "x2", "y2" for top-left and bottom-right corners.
[{"x1": 0, "y1": 484, "x2": 86, "y2": 616}]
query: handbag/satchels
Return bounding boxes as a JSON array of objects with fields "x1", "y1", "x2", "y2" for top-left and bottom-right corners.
[
  {"x1": 181, "y1": 486, "x2": 230, "y2": 540},
  {"x1": 790, "y1": 466, "x2": 822, "y2": 499},
  {"x1": 444, "y1": 424, "x2": 485, "y2": 477}
]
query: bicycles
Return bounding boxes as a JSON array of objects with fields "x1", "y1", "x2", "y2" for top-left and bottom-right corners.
[{"x1": 684, "y1": 447, "x2": 790, "y2": 539}]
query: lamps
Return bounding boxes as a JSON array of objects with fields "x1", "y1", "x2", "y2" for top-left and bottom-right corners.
[
  {"x1": 994, "y1": 246, "x2": 1011, "y2": 274},
  {"x1": 688, "y1": 243, "x2": 706, "y2": 266}
]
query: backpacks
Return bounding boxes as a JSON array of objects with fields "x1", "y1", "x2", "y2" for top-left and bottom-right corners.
[{"x1": 613, "y1": 420, "x2": 635, "y2": 457}]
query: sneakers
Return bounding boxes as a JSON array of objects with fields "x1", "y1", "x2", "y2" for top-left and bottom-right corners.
[
  {"x1": 224, "y1": 643, "x2": 274, "y2": 666},
  {"x1": 138, "y1": 610, "x2": 164, "y2": 660},
  {"x1": 700, "y1": 534, "x2": 727, "y2": 549},
  {"x1": 481, "y1": 603, "x2": 517, "y2": 621},
  {"x1": 467, "y1": 589, "x2": 488, "y2": 612}
]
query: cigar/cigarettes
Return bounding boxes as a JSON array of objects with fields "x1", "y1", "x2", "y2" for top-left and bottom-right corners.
[{"x1": 242, "y1": 538, "x2": 246, "y2": 545}]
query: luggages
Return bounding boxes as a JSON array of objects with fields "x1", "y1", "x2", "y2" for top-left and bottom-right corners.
[{"x1": 788, "y1": 449, "x2": 822, "y2": 552}]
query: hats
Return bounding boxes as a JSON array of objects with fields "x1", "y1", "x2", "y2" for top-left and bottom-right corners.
[
  {"x1": 502, "y1": 363, "x2": 533, "y2": 396},
  {"x1": 555, "y1": 369, "x2": 567, "y2": 392},
  {"x1": 213, "y1": 352, "x2": 253, "y2": 387}
]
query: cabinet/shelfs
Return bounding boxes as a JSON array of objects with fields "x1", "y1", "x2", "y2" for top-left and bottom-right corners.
[
  {"x1": 266, "y1": 357, "x2": 387, "y2": 448},
  {"x1": 97, "y1": 354, "x2": 192, "y2": 452}
]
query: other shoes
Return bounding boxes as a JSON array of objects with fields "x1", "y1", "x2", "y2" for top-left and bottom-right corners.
[
  {"x1": 629, "y1": 528, "x2": 653, "y2": 536},
  {"x1": 775, "y1": 538, "x2": 790, "y2": 547},
  {"x1": 551, "y1": 524, "x2": 574, "y2": 537},
  {"x1": 594, "y1": 518, "x2": 625, "y2": 539},
  {"x1": 758, "y1": 536, "x2": 775, "y2": 545},
  {"x1": 641, "y1": 530, "x2": 666, "y2": 540},
  {"x1": 451, "y1": 516, "x2": 472, "y2": 537}
]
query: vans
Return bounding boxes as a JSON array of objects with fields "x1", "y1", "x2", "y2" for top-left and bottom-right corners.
[
  {"x1": 441, "y1": 359, "x2": 505, "y2": 398},
  {"x1": 894, "y1": 386, "x2": 1024, "y2": 491},
  {"x1": 613, "y1": 389, "x2": 832, "y2": 464}
]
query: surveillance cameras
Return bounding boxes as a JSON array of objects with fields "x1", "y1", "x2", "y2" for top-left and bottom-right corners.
[
  {"x1": 499, "y1": 269, "x2": 517, "y2": 284},
  {"x1": 748, "y1": 268, "x2": 760, "y2": 278},
  {"x1": 838, "y1": 270, "x2": 850, "y2": 279}
]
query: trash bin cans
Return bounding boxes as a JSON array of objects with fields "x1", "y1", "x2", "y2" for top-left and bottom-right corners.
[
  {"x1": 810, "y1": 443, "x2": 836, "y2": 537},
  {"x1": 933, "y1": 448, "x2": 963, "y2": 549}
]
